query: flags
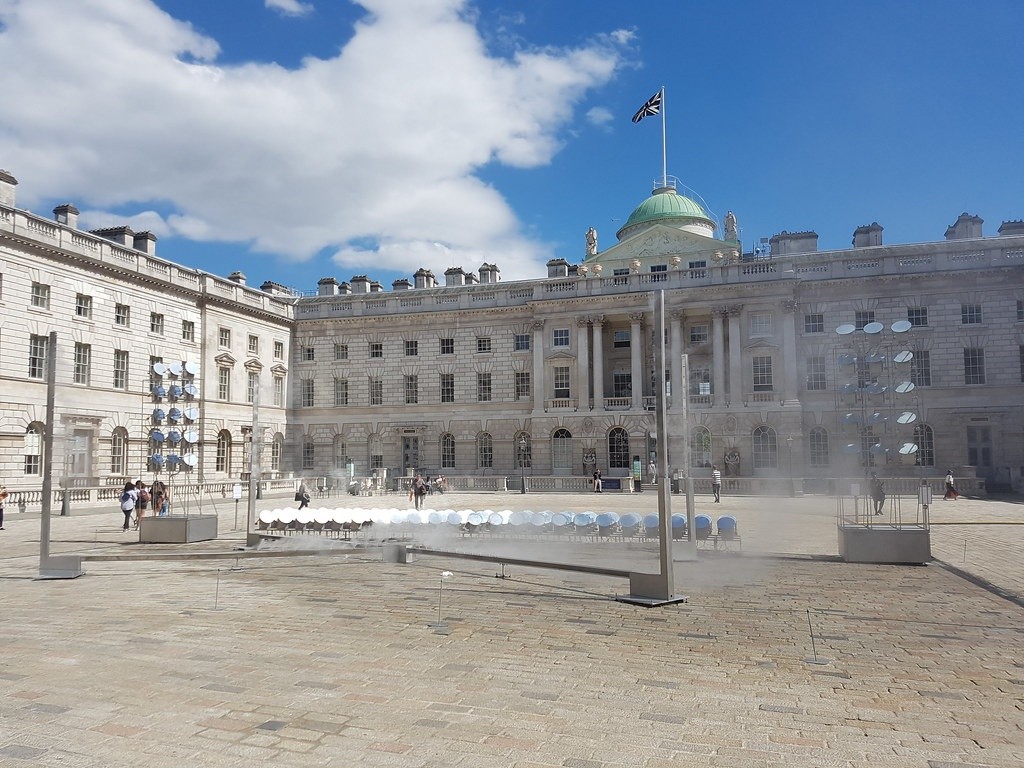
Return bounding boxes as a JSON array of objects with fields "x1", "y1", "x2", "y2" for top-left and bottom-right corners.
[{"x1": 632, "y1": 89, "x2": 662, "y2": 123}]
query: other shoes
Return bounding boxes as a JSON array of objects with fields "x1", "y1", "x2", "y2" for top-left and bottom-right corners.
[
  {"x1": 714, "y1": 498, "x2": 720, "y2": 503},
  {"x1": 943, "y1": 497, "x2": 948, "y2": 501},
  {"x1": 878, "y1": 510, "x2": 883, "y2": 515},
  {"x1": 123, "y1": 529, "x2": 130, "y2": 532}
]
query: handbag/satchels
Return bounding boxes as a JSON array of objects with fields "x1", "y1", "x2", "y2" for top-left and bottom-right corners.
[{"x1": 295, "y1": 492, "x2": 303, "y2": 501}]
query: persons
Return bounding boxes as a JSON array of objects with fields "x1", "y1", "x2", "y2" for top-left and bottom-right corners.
[
  {"x1": 298, "y1": 478, "x2": 310, "y2": 511},
  {"x1": 712, "y1": 465, "x2": 721, "y2": 503},
  {"x1": 350, "y1": 478, "x2": 359, "y2": 497},
  {"x1": 411, "y1": 473, "x2": 426, "y2": 510},
  {"x1": 943, "y1": 470, "x2": 958, "y2": 501},
  {"x1": 437, "y1": 475, "x2": 445, "y2": 495},
  {"x1": 870, "y1": 472, "x2": 885, "y2": 515},
  {"x1": 424, "y1": 477, "x2": 433, "y2": 496},
  {"x1": 592, "y1": 469, "x2": 602, "y2": 493},
  {"x1": 0, "y1": 485, "x2": 9, "y2": 530},
  {"x1": 649, "y1": 461, "x2": 656, "y2": 485},
  {"x1": 120, "y1": 480, "x2": 168, "y2": 533}
]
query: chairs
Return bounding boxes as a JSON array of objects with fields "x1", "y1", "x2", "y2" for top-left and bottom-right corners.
[{"x1": 257, "y1": 509, "x2": 741, "y2": 554}]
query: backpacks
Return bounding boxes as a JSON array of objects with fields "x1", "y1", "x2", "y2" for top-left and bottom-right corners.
[{"x1": 121, "y1": 490, "x2": 130, "y2": 502}]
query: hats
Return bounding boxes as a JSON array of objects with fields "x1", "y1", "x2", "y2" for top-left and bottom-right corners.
[{"x1": 871, "y1": 471, "x2": 877, "y2": 476}]
